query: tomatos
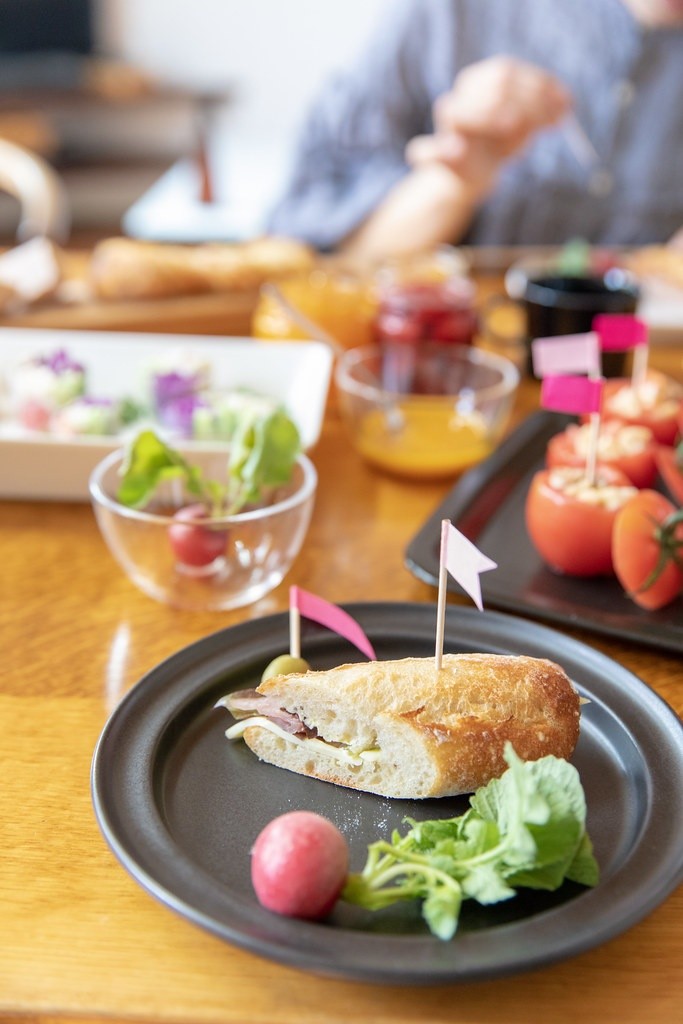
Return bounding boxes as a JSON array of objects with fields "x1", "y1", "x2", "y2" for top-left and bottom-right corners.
[{"x1": 526, "y1": 370, "x2": 683, "y2": 609}]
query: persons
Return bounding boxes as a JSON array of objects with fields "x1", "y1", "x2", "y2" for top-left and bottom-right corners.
[{"x1": 265, "y1": 1, "x2": 683, "y2": 259}]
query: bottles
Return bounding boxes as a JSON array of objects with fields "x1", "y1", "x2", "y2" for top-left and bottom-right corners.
[{"x1": 367, "y1": 260, "x2": 478, "y2": 392}]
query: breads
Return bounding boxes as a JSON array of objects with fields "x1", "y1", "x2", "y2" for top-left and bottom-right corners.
[{"x1": 213, "y1": 651, "x2": 580, "y2": 800}]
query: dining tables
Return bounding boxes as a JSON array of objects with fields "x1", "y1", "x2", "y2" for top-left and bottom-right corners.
[{"x1": 0, "y1": 245, "x2": 683, "y2": 1024}]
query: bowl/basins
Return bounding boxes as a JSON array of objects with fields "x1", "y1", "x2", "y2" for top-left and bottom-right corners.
[
  {"x1": 89, "y1": 441, "x2": 319, "y2": 610},
  {"x1": 337, "y1": 338, "x2": 521, "y2": 482}
]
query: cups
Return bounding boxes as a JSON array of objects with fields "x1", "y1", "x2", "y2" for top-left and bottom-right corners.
[{"x1": 524, "y1": 272, "x2": 638, "y2": 380}]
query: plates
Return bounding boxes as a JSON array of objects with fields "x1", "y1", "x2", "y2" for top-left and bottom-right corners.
[
  {"x1": 89, "y1": 602, "x2": 682, "y2": 988},
  {"x1": 404, "y1": 410, "x2": 682, "y2": 650},
  {"x1": 1, "y1": 327, "x2": 336, "y2": 502}
]
